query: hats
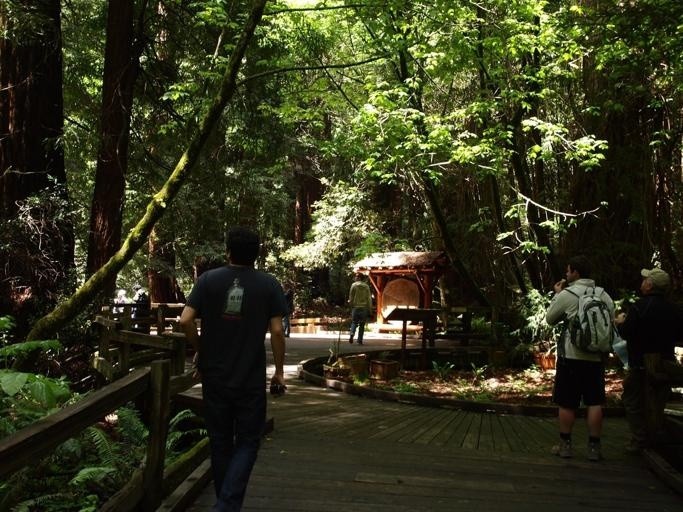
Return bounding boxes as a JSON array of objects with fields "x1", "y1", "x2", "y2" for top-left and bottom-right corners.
[{"x1": 640, "y1": 269, "x2": 671, "y2": 287}]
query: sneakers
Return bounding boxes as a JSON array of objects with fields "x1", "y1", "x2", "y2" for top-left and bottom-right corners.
[
  {"x1": 624, "y1": 432, "x2": 671, "y2": 455},
  {"x1": 349, "y1": 338, "x2": 363, "y2": 345},
  {"x1": 551, "y1": 439, "x2": 576, "y2": 457},
  {"x1": 587, "y1": 441, "x2": 600, "y2": 461}
]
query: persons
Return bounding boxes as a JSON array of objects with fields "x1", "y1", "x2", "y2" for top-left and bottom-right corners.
[
  {"x1": 614, "y1": 268, "x2": 674, "y2": 456},
  {"x1": 181, "y1": 227, "x2": 287, "y2": 512},
  {"x1": 282, "y1": 283, "x2": 294, "y2": 338},
  {"x1": 347, "y1": 272, "x2": 372, "y2": 345},
  {"x1": 546, "y1": 256, "x2": 615, "y2": 460},
  {"x1": 132, "y1": 285, "x2": 146, "y2": 301},
  {"x1": 114, "y1": 289, "x2": 130, "y2": 313}
]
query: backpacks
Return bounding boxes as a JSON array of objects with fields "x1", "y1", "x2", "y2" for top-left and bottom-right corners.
[{"x1": 564, "y1": 286, "x2": 614, "y2": 353}]
